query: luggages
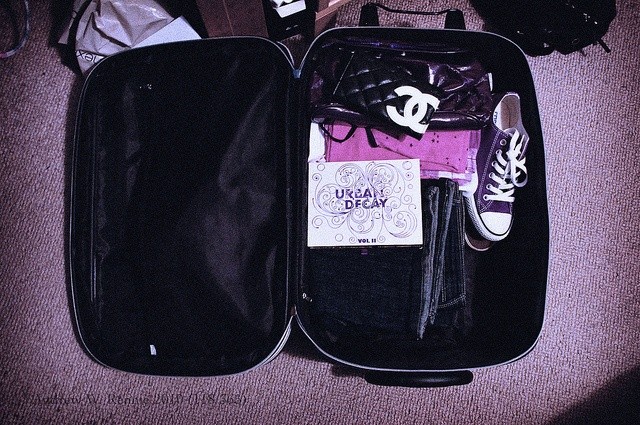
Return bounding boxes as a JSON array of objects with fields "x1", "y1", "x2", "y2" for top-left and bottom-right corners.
[{"x1": 62, "y1": 26, "x2": 551, "y2": 388}]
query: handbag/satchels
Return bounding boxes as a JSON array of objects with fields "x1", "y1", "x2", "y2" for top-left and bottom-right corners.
[
  {"x1": 330, "y1": 46, "x2": 445, "y2": 141},
  {"x1": 308, "y1": 35, "x2": 495, "y2": 130}
]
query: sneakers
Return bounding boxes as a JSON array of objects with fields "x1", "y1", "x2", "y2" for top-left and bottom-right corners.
[{"x1": 463, "y1": 89, "x2": 530, "y2": 242}]
